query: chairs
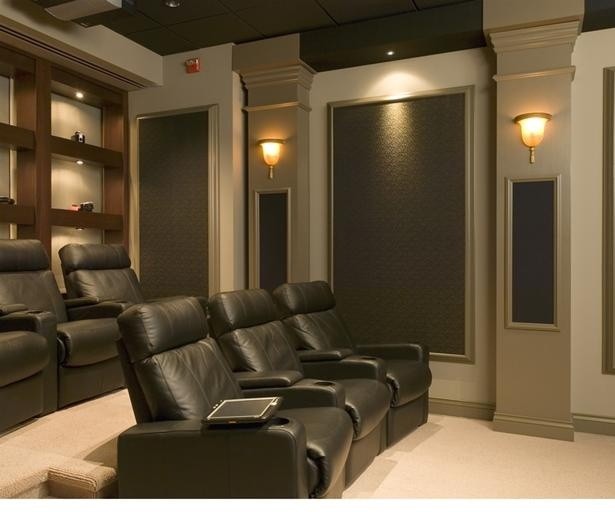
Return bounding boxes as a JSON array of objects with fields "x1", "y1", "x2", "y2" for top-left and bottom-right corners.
[
  {"x1": 1, "y1": 303, "x2": 56, "y2": 436},
  {"x1": 57, "y1": 243, "x2": 183, "y2": 302},
  {"x1": 0, "y1": 240, "x2": 131, "y2": 408},
  {"x1": 272, "y1": 280, "x2": 433, "y2": 448},
  {"x1": 116, "y1": 292, "x2": 356, "y2": 500},
  {"x1": 203, "y1": 291, "x2": 395, "y2": 491}
]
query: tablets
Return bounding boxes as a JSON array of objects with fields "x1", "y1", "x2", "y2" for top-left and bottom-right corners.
[{"x1": 200, "y1": 395, "x2": 284, "y2": 426}]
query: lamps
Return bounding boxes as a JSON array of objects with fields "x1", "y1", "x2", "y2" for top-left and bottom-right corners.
[
  {"x1": 256, "y1": 137, "x2": 286, "y2": 182},
  {"x1": 511, "y1": 111, "x2": 550, "y2": 164}
]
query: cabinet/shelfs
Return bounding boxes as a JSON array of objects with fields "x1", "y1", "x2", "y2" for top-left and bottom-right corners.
[{"x1": 0, "y1": 41, "x2": 130, "y2": 297}]
